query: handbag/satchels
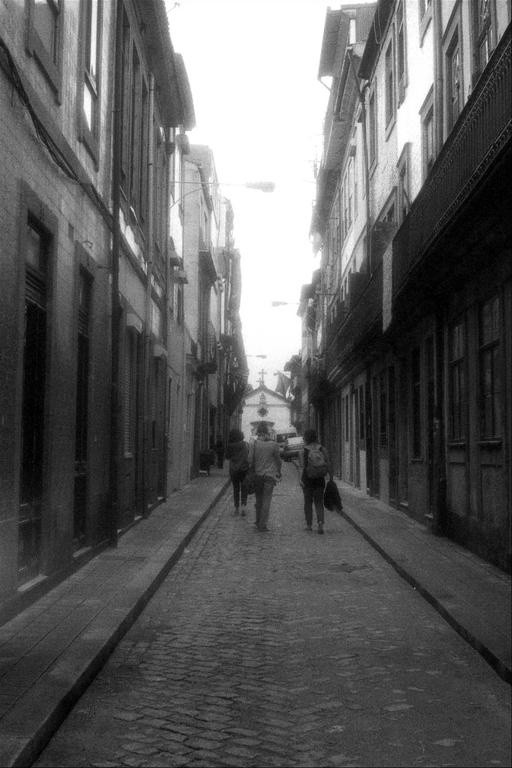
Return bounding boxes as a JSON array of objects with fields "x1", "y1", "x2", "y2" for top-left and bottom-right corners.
[
  {"x1": 324, "y1": 478, "x2": 343, "y2": 512},
  {"x1": 244, "y1": 468, "x2": 257, "y2": 494}
]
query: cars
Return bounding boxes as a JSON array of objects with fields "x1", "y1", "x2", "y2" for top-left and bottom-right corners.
[{"x1": 276, "y1": 432, "x2": 305, "y2": 462}]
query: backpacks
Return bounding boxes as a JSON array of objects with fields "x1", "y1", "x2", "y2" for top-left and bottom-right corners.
[{"x1": 304, "y1": 444, "x2": 328, "y2": 479}]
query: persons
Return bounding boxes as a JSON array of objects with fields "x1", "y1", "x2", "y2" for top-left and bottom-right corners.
[
  {"x1": 244, "y1": 422, "x2": 283, "y2": 531},
  {"x1": 295, "y1": 430, "x2": 334, "y2": 534},
  {"x1": 226, "y1": 428, "x2": 251, "y2": 516}
]
